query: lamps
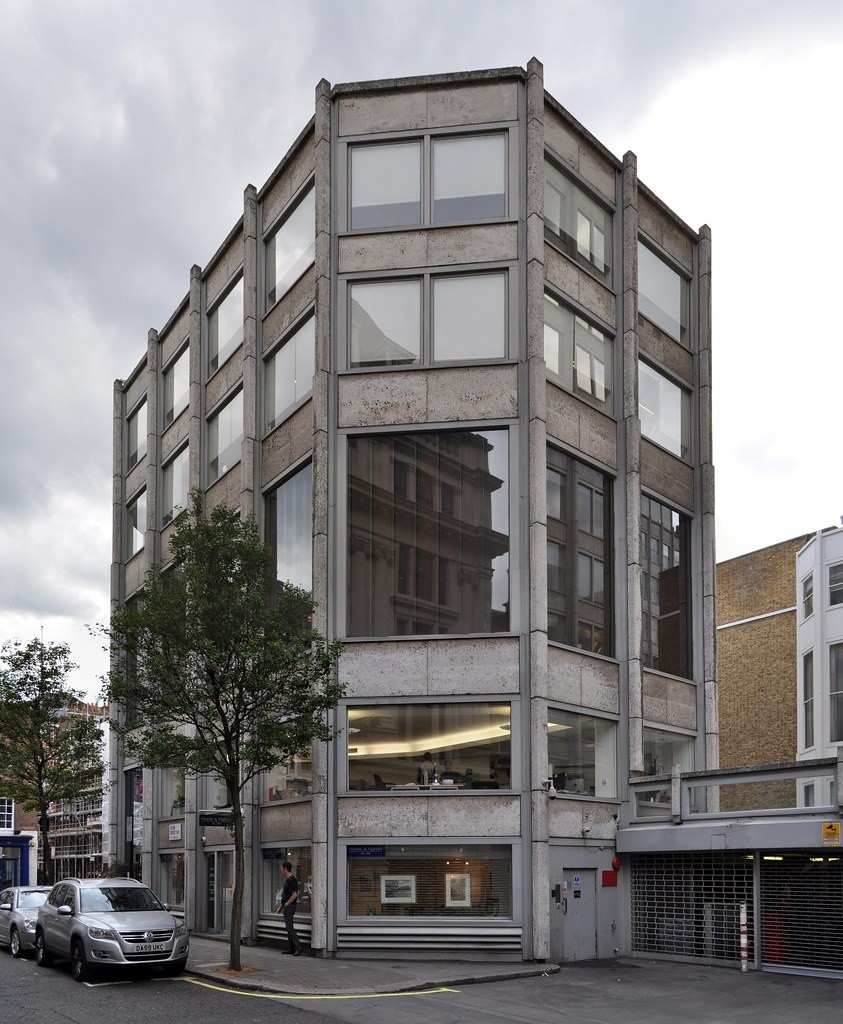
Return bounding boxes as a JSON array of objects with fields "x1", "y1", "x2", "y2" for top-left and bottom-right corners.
[{"x1": 542, "y1": 779, "x2": 557, "y2": 801}]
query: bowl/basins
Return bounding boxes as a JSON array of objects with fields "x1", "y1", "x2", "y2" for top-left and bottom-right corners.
[{"x1": 442, "y1": 779, "x2": 454, "y2": 785}]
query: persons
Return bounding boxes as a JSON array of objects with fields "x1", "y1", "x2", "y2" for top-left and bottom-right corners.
[
  {"x1": 416, "y1": 753, "x2": 499, "y2": 790},
  {"x1": 275, "y1": 861, "x2": 304, "y2": 956}
]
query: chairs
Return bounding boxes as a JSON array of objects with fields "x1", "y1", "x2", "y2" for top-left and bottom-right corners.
[{"x1": 373, "y1": 774, "x2": 397, "y2": 791}]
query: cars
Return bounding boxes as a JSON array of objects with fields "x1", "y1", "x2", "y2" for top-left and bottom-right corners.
[{"x1": 0, "y1": 886, "x2": 55, "y2": 958}]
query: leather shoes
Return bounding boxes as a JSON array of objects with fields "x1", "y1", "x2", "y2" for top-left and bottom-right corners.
[
  {"x1": 293, "y1": 950, "x2": 302, "y2": 956},
  {"x1": 281, "y1": 949, "x2": 293, "y2": 954}
]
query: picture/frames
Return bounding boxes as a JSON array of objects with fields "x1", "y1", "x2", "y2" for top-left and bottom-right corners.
[
  {"x1": 379, "y1": 873, "x2": 418, "y2": 905},
  {"x1": 443, "y1": 872, "x2": 472, "y2": 911}
]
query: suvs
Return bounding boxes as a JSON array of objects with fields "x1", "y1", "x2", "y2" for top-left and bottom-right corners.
[{"x1": 35, "y1": 876, "x2": 190, "y2": 982}]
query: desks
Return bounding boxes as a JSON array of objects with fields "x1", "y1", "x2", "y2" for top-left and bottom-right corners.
[{"x1": 386, "y1": 784, "x2": 465, "y2": 790}]
